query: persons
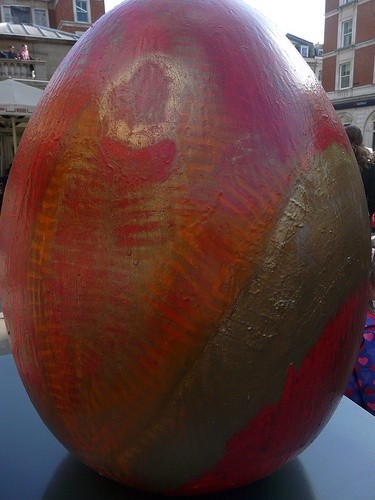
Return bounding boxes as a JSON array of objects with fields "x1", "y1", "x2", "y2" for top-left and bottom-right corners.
[
  {"x1": 0, "y1": 50, "x2": 8, "y2": 59},
  {"x1": 345, "y1": 125, "x2": 375, "y2": 218},
  {"x1": 18, "y1": 44, "x2": 30, "y2": 60},
  {"x1": 6, "y1": 45, "x2": 18, "y2": 79}
]
